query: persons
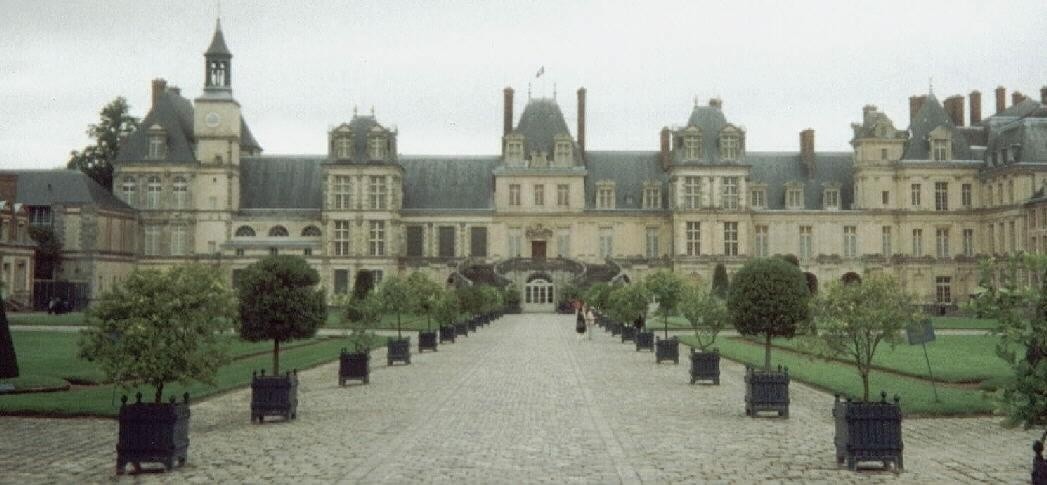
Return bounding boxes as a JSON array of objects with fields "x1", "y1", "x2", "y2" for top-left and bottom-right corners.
[{"x1": 575, "y1": 305, "x2": 596, "y2": 338}]
